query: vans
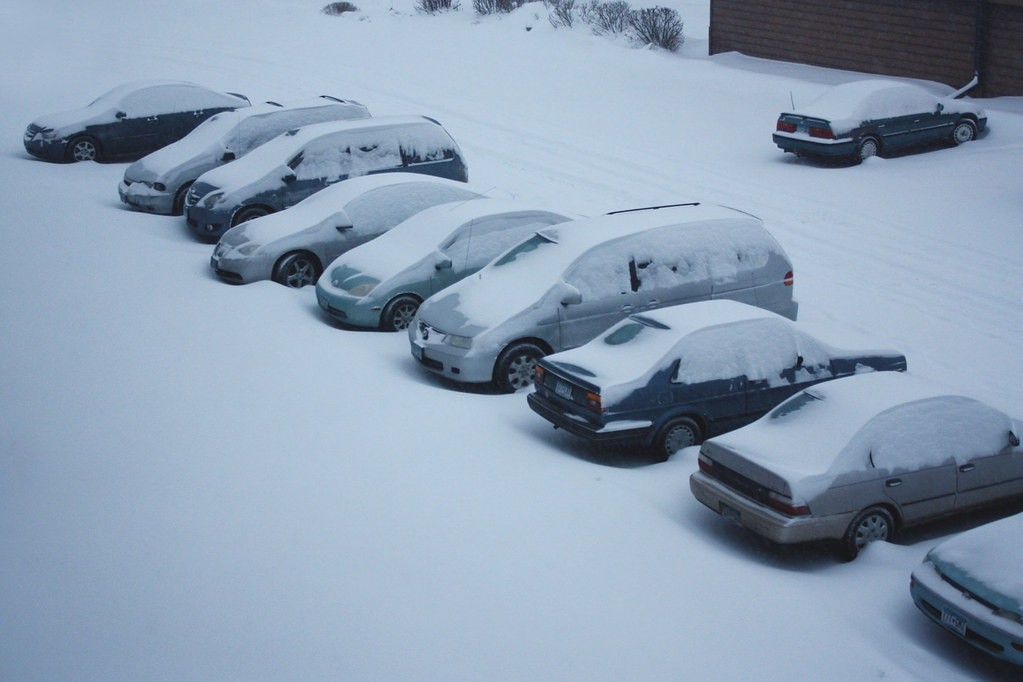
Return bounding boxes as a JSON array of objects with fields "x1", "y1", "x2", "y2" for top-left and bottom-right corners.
[
  {"x1": 181, "y1": 111, "x2": 469, "y2": 244},
  {"x1": 407, "y1": 199, "x2": 801, "y2": 398}
]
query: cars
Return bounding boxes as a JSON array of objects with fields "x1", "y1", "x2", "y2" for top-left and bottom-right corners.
[
  {"x1": 528, "y1": 298, "x2": 908, "y2": 467},
  {"x1": 772, "y1": 79, "x2": 988, "y2": 167},
  {"x1": 24, "y1": 80, "x2": 252, "y2": 165},
  {"x1": 908, "y1": 509, "x2": 1022, "y2": 670},
  {"x1": 315, "y1": 200, "x2": 579, "y2": 331},
  {"x1": 686, "y1": 370, "x2": 1023, "y2": 561},
  {"x1": 208, "y1": 173, "x2": 491, "y2": 290}
]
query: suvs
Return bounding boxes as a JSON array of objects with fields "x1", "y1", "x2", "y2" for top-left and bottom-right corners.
[{"x1": 117, "y1": 91, "x2": 376, "y2": 214}]
